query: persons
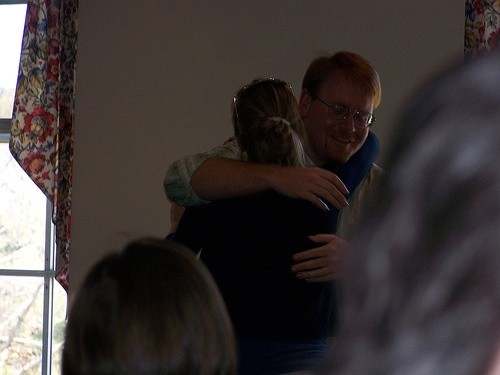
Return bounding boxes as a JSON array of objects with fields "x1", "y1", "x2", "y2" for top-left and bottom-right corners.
[
  {"x1": 62, "y1": 236, "x2": 236, "y2": 375},
  {"x1": 164, "y1": 49, "x2": 385, "y2": 285},
  {"x1": 162, "y1": 77, "x2": 337, "y2": 374}
]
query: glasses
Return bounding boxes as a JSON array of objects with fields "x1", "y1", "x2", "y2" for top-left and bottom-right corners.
[{"x1": 313, "y1": 92, "x2": 376, "y2": 130}]
